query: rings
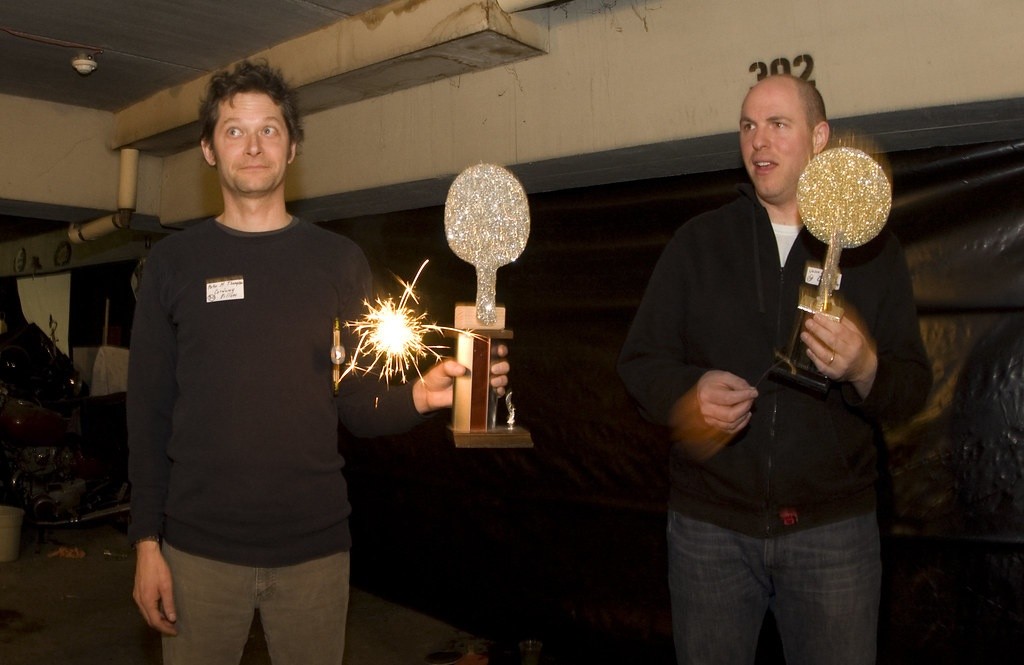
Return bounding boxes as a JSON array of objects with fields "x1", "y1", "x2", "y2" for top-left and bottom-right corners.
[{"x1": 826, "y1": 353, "x2": 836, "y2": 366}]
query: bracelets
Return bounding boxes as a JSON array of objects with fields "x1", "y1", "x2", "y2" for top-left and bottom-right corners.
[{"x1": 132, "y1": 536, "x2": 160, "y2": 552}]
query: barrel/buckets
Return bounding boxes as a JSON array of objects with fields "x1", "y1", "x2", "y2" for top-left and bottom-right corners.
[{"x1": 0, "y1": 506, "x2": 24, "y2": 562}]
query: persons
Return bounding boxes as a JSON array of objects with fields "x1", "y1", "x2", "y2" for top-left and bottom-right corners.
[
  {"x1": 614, "y1": 76, "x2": 935, "y2": 664},
  {"x1": 126, "y1": 52, "x2": 510, "y2": 665}
]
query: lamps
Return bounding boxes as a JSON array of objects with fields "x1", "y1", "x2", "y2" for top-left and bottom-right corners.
[
  {"x1": 438, "y1": 164, "x2": 537, "y2": 450},
  {"x1": 776, "y1": 149, "x2": 893, "y2": 392}
]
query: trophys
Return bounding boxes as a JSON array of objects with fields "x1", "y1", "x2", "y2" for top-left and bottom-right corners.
[
  {"x1": 441, "y1": 163, "x2": 539, "y2": 449},
  {"x1": 766, "y1": 139, "x2": 895, "y2": 399}
]
